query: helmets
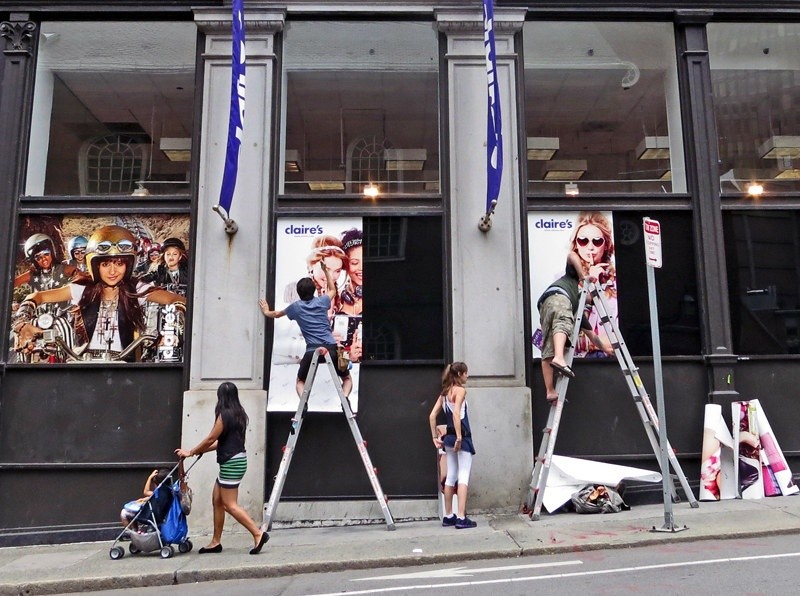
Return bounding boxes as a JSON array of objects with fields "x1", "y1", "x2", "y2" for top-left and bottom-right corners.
[
  {"x1": 24, "y1": 234, "x2": 56, "y2": 266},
  {"x1": 160, "y1": 238, "x2": 185, "y2": 254},
  {"x1": 68, "y1": 236, "x2": 88, "y2": 259},
  {"x1": 147, "y1": 242, "x2": 162, "y2": 255},
  {"x1": 86, "y1": 225, "x2": 136, "y2": 281}
]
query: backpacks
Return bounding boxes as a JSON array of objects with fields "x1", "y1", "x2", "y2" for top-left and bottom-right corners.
[{"x1": 159, "y1": 497, "x2": 188, "y2": 545}]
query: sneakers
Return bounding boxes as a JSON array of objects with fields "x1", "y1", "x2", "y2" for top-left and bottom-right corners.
[
  {"x1": 455, "y1": 516, "x2": 477, "y2": 529},
  {"x1": 441, "y1": 513, "x2": 457, "y2": 527}
]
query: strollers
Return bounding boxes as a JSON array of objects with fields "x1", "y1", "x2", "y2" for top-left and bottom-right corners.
[{"x1": 109, "y1": 449, "x2": 204, "y2": 559}]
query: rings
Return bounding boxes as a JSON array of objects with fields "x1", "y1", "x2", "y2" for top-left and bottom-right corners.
[{"x1": 16, "y1": 338, "x2": 36, "y2": 352}]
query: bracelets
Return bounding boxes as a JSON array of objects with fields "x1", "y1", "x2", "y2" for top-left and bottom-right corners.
[
  {"x1": 434, "y1": 437, "x2": 438, "y2": 438},
  {"x1": 168, "y1": 301, "x2": 187, "y2": 336},
  {"x1": 13, "y1": 299, "x2": 37, "y2": 334}
]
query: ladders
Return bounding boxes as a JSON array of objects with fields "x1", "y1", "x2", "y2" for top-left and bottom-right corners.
[
  {"x1": 523, "y1": 279, "x2": 699, "y2": 521},
  {"x1": 261, "y1": 347, "x2": 396, "y2": 532}
]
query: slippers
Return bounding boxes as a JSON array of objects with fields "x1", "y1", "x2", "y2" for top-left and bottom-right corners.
[
  {"x1": 547, "y1": 395, "x2": 568, "y2": 403},
  {"x1": 550, "y1": 361, "x2": 576, "y2": 379}
]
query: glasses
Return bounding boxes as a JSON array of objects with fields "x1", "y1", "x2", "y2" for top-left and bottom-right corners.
[
  {"x1": 34, "y1": 249, "x2": 51, "y2": 261},
  {"x1": 576, "y1": 237, "x2": 605, "y2": 247},
  {"x1": 74, "y1": 250, "x2": 86, "y2": 254},
  {"x1": 85, "y1": 239, "x2": 137, "y2": 256}
]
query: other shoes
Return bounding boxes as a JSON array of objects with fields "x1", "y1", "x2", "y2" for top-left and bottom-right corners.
[
  {"x1": 341, "y1": 399, "x2": 353, "y2": 414},
  {"x1": 119, "y1": 534, "x2": 132, "y2": 541},
  {"x1": 249, "y1": 532, "x2": 269, "y2": 554},
  {"x1": 300, "y1": 402, "x2": 308, "y2": 419},
  {"x1": 198, "y1": 543, "x2": 222, "y2": 554}
]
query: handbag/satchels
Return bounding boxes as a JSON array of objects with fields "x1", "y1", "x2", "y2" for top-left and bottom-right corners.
[
  {"x1": 572, "y1": 483, "x2": 631, "y2": 514},
  {"x1": 178, "y1": 481, "x2": 193, "y2": 515},
  {"x1": 337, "y1": 350, "x2": 350, "y2": 372}
]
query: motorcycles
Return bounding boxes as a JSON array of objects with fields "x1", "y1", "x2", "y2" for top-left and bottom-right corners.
[{"x1": 31, "y1": 302, "x2": 78, "y2": 361}]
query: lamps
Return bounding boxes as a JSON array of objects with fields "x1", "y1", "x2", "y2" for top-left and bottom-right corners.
[
  {"x1": 743, "y1": 181, "x2": 763, "y2": 195},
  {"x1": 525, "y1": 137, "x2": 559, "y2": 161},
  {"x1": 541, "y1": 160, "x2": 587, "y2": 180},
  {"x1": 384, "y1": 148, "x2": 427, "y2": 170},
  {"x1": 364, "y1": 182, "x2": 380, "y2": 195},
  {"x1": 284, "y1": 150, "x2": 303, "y2": 173},
  {"x1": 133, "y1": 183, "x2": 151, "y2": 196},
  {"x1": 304, "y1": 170, "x2": 346, "y2": 189},
  {"x1": 565, "y1": 182, "x2": 579, "y2": 194},
  {"x1": 634, "y1": 136, "x2": 669, "y2": 160},
  {"x1": 758, "y1": 136, "x2": 800, "y2": 159},
  {"x1": 160, "y1": 138, "x2": 192, "y2": 161}
]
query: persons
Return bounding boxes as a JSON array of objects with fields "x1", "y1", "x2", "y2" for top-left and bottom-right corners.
[
  {"x1": 9, "y1": 226, "x2": 187, "y2": 364},
  {"x1": 134, "y1": 233, "x2": 161, "y2": 278},
  {"x1": 429, "y1": 362, "x2": 477, "y2": 528},
  {"x1": 259, "y1": 260, "x2": 353, "y2": 416},
  {"x1": 118, "y1": 469, "x2": 171, "y2": 543},
  {"x1": 572, "y1": 211, "x2": 617, "y2": 358},
  {"x1": 283, "y1": 228, "x2": 362, "y2": 364},
  {"x1": 173, "y1": 382, "x2": 270, "y2": 554},
  {"x1": 138, "y1": 239, "x2": 188, "y2": 298},
  {"x1": 537, "y1": 252, "x2": 616, "y2": 404},
  {"x1": 14, "y1": 234, "x2": 87, "y2": 351},
  {"x1": 62, "y1": 236, "x2": 88, "y2": 273}
]
